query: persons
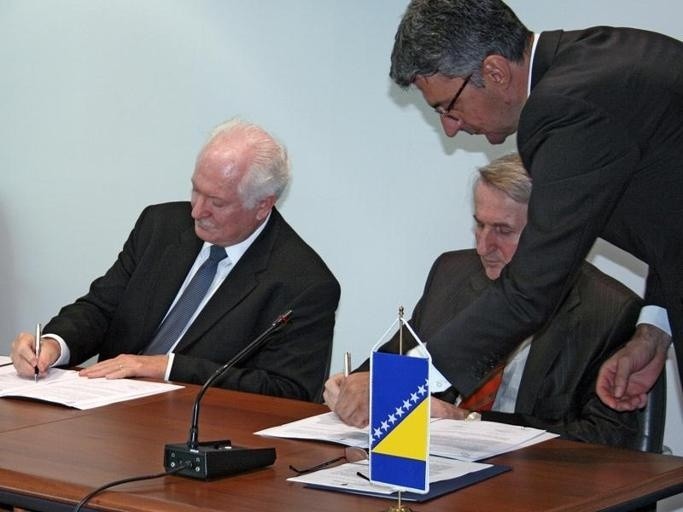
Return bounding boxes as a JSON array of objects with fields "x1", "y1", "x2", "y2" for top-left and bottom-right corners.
[
  {"x1": 331, "y1": 0, "x2": 683, "y2": 438},
  {"x1": 6, "y1": 113, "x2": 341, "y2": 407},
  {"x1": 317, "y1": 151, "x2": 645, "y2": 447}
]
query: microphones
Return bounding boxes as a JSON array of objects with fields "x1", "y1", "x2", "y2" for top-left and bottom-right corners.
[{"x1": 164, "y1": 306, "x2": 293, "y2": 481}]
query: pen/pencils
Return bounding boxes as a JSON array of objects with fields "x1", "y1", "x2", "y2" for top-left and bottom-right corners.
[
  {"x1": 35, "y1": 323, "x2": 41, "y2": 383},
  {"x1": 344, "y1": 352, "x2": 349, "y2": 380}
]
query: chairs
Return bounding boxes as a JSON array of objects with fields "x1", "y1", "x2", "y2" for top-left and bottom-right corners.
[{"x1": 616, "y1": 351, "x2": 671, "y2": 512}]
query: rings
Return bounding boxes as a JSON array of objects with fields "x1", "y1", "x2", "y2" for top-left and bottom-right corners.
[{"x1": 118, "y1": 362, "x2": 123, "y2": 370}]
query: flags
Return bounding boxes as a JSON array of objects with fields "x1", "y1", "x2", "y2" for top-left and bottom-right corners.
[{"x1": 369, "y1": 349, "x2": 428, "y2": 493}]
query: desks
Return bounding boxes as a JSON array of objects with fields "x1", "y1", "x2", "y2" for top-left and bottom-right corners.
[{"x1": 0, "y1": 359, "x2": 681, "y2": 512}]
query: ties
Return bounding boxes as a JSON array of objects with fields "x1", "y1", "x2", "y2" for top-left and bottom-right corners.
[
  {"x1": 455, "y1": 366, "x2": 506, "y2": 412},
  {"x1": 141, "y1": 244, "x2": 229, "y2": 357}
]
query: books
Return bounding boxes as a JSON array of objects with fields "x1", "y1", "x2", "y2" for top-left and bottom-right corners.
[
  {"x1": 0, "y1": 356, "x2": 190, "y2": 413},
  {"x1": 282, "y1": 451, "x2": 511, "y2": 505},
  {"x1": 251, "y1": 391, "x2": 562, "y2": 465}
]
